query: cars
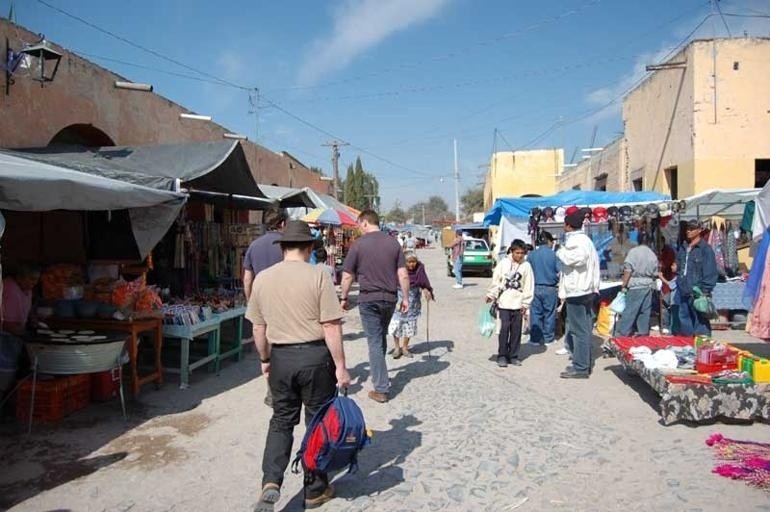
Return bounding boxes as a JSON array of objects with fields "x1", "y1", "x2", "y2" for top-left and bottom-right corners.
[{"x1": 445, "y1": 236, "x2": 496, "y2": 276}]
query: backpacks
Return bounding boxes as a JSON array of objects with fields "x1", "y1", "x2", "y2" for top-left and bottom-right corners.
[{"x1": 292, "y1": 381, "x2": 371, "y2": 484}]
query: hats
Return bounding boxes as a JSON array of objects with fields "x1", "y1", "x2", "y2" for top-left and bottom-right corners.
[
  {"x1": 686, "y1": 219, "x2": 703, "y2": 230},
  {"x1": 618, "y1": 206, "x2": 633, "y2": 225},
  {"x1": 552, "y1": 207, "x2": 567, "y2": 222},
  {"x1": 272, "y1": 221, "x2": 325, "y2": 250},
  {"x1": 580, "y1": 207, "x2": 592, "y2": 222},
  {"x1": 566, "y1": 207, "x2": 579, "y2": 217},
  {"x1": 658, "y1": 203, "x2": 674, "y2": 218},
  {"x1": 594, "y1": 207, "x2": 610, "y2": 224},
  {"x1": 535, "y1": 231, "x2": 558, "y2": 246},
  {"x1": 607, "y1": 207, "x2": 621, "y2": 224},
  {"x1": 647, "y1": 204, "x2": 661, "y2": 220},
  {"x1": 565, "y1": 211, "x2": 586, "y2": 229},
  {"x1": 541, "y1": 207, "x2": 556, "y2": 223},
  {"x1": 632, "y1": 206, "x2": 647, "y2": 222}
]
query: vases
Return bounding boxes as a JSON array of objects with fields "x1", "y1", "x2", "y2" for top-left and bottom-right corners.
[{"x1": 96, "y1": 305, "x2": 115, "y2": 320}]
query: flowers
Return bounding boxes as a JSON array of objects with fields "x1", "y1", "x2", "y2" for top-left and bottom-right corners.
[
  {"x1": 113, "y1": 278, "x2": 164, "y2": 323},
  {"x1": 41, "y1": 263, "x2": 81, "y2": 301},
  {"x1": 88, "y1": 276, "x2": 117, "y2": 305}
]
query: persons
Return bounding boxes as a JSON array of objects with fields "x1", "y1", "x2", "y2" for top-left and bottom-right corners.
[
  {"x1": 616, "y1": 229, "x2": 658, "y2": 338},
  {"x1": 403, "y1": 231, "x2": 419, "y2": 252},
  {"x1": 0, "y1": 259, "x2": 41, "y2": 394},
  {"x1": 341, "y1": 210, "x2": 409, "y2": 403},
  {"x1": 659, "y1": 261, "x2": 679, "y2": 336},
  {"x1": 672, "y1": 220, "x2": 717, "y2": 337},
  {"x1": 389, "y1": 251, "x2": 435, "y2": 359},
  {"x1": 446, "y1": 230, "x2": 464, "y2": 289},
  {"x1": 552, "y1": 214, "x2": 600, "y2": 378},
  {"x1": 313, "y1": 248, "x2": 337, "y2": 286},
  {"x1": 242, "y1": 209, "x2": 289, "y2": 304},
  {"x1": 245, "y1": 221, "x2": 349, "y2": 511},
  {"x1": 485, "y1": 238, "x2": 535, "y2": 367},
  {"x1": 555, "y1": 303, "x2": 598, "y2": 356}
]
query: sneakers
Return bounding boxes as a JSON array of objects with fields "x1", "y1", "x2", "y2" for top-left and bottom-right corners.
[
  {"x1": 511, "y1": 357, "x2": 524, "y2": 366},
  {"x1": 544, "y1": 339, "x2": 557, "y2": 346},
  {"x1": 499, "y1": 357, "x2": 510, "y2": 368},
  {"x1": 453, "y1": 283, "x2": 463, "y2": 289},
  {"x1": 529, "y1": 341, "x2": 541, "y2": 346}
]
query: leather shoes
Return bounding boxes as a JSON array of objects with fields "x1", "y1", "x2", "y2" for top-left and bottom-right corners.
[
  {"x1": 568, "y1": 365, "x2": 578, "y2": 370},
  {"x1": 560, "y1": 370, "x2": 591, "y2": 380},
  {"x1": 393, "y1": 349, "x2": 403, "y2": 360},
  {"x1": 304, "y1": 486, "x2": 336, "y2": 509},
  {"x1": 255, "y1": 482, "x2": 280, "y2": 512},
  {"x1": 369, "y1": 391, "x2": 390, "y2": 403},
  {"x1": 402, "y1": 350, "x2": 414, "y2": 360}
]
